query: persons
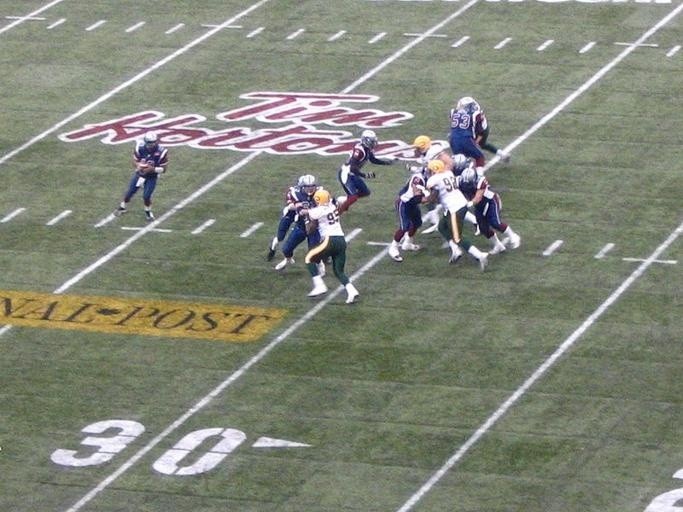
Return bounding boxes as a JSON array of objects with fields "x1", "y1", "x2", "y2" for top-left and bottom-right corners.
[
  {"x1": 387, "y1": 166, "x2": 428, "y2": 262},
  {"x1": 473, "y1": 108, "x2": 511, "y2": 161},
  {"x1": 450, "y1": 153, "x2": 485, "y2": 176},
  {"x1": 267, "y1": 176, "x2": 333, "y2": 265},
  {"x1": 274, "y1": 174, "x2": 326, "y2": 278},
  {"x1": 115, "y1": 133, "x2": 169, "y2": 221},
  {"x1": 454, "y1": 167, "x2": 521, "y2": 255},
  {"x1": 414, "y1": 135, "x2": 481, "y2": 237},
  {"x1": 299, "y1": 189, "x2": 359, "y2": 304},
  {"x1": 426, "y1": 159, "x2": 489, "y2": 273},
  {"x1": 448, "y1": 97, "x2": 485, "y2": 188},
  {"x1": 336, "y1": 128, "x2": 399, "y2": 214}
]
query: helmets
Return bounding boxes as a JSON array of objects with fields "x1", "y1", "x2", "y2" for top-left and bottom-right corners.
[
  {"x1": 297, "y1": 175, "x2": 305, "y2": 186},
  {"x1": 360, "y1": 130, "x2": 378, "y2": 150},
  {"x1": 303, "y1": 174, "x2": 317, "y2": 187},
  {"x1": 453, "y1": 154, "x2": 466, "y2": 173},
  {"x1": 458, "y1": 96, "x2": 476, "y2": 112},
  {"x1": 144, "y1": 130, "x2": 158, "y2": 143},
  {"x1": 413, "y1": 135, "x2": 432, "y2": 154},
  {"x1": 459, "y1": 167, "x2": 477, "y2": 190},
  {"x1": 313, "y1": 190, "x2": 331, "y2": 205},
  {"x1": 428, "y1": 160, "x2": 444, "y2": 173}
]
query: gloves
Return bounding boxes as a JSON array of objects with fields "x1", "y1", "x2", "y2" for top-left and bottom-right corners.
[
  {"x1": 415, "y1": 184, "x2": 430, "y2": 196},
  {"x1": 363, "y1": 171, "x2": 377, "y2": 180}
]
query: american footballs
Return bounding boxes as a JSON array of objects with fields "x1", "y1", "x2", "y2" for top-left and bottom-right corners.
[{"x1": 138, "y1": 162, "x2": 148, "y2": 176}]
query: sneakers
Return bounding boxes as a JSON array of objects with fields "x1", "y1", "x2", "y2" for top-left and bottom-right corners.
[
  {"x1": 473, "y1": 222, "x2": 482, "y2": 236},
  {"x1": 267, "y1": 247, "x2": 277, "y2": 261},
  {"x1": 345, "y1": 289, "x2": 360, "y2": 304},
  {"x1": 477, "y1": 251, "x2": 489, "y2": 271},
  {"x1": 388, "y1": 247, "x2": 404, "y2": 262},
  {"x1": 401, "y1": 242, "x2": 421, "y2": 251},
  {"x1": 488, "y1": 242, "x2": 506, "y2": 255},
  {"x1": 307, "y1": 284, "x2": 328, "y2": 298},
  {"x1": 448, "y1": 249, "x2": 464, "y2": 264},
  {"x1": 501, "y1": 151, "x2": 512, "y2": 163},
  {"x1": 316, "y1": 260, "x2": 326, "y2": 277},
  {"x1": 275, "y1": 256, "x2": 296, "y2": 271},
  {"x1": 144, "y1": 209, "x2": 156, "y2": 221},
  {"x1": 117, "y1": 205, "x2": 127, "y2": 213},
  {"x1": 509, "y1": 233, "x2": 521, "y2": 250}
]
